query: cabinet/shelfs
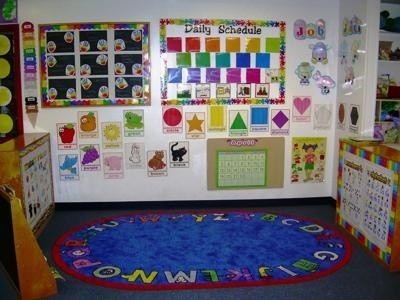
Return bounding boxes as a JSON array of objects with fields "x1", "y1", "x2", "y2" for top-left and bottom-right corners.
[{"x1": 336, "y1": 0, "x2": 400, "y2": 138}]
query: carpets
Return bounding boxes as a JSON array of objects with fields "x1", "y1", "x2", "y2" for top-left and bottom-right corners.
[{"x1": 53, "y1": 210, "x2": 352, "y2": 291}]
